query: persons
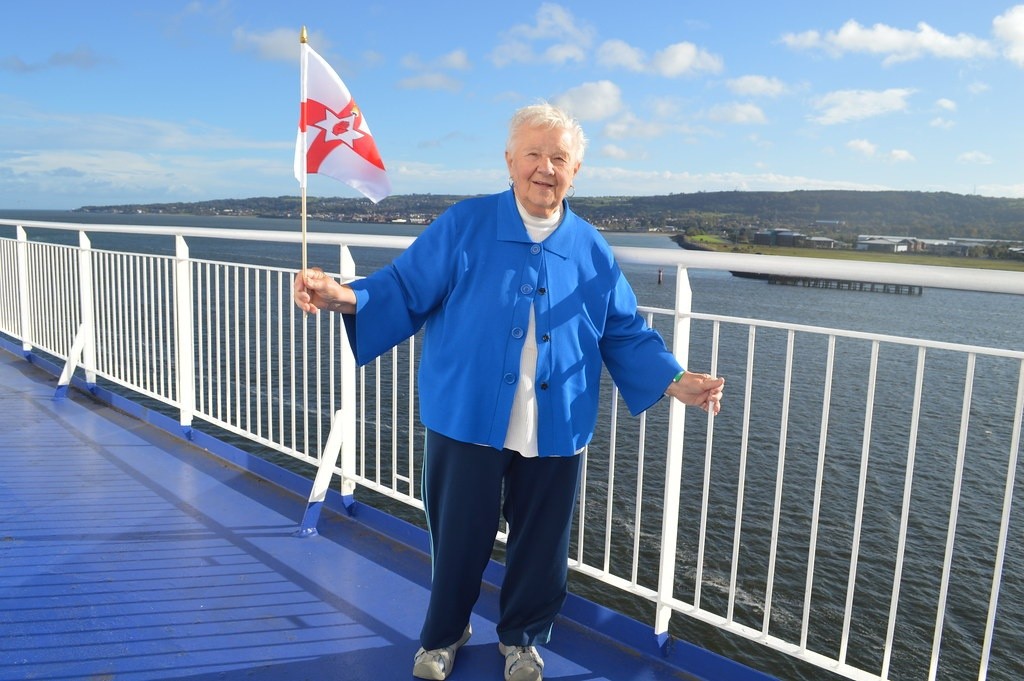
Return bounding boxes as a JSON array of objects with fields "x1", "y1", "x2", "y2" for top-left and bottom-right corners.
[{"x1": 295, "y1": 105, "x2": 725, "y2": 681}]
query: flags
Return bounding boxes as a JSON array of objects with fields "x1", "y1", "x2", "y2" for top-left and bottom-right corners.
[{"x1": 294, "y1": 45, "x2": 391, "y2": 203}]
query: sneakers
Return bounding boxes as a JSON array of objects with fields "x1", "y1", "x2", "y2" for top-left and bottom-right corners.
[
  {"x1": 498, "y1": 640, "x2": 544, "y2": 681},
  {"x1": 413, "y1": 621, "x2": 472, "y2": 681}
]
